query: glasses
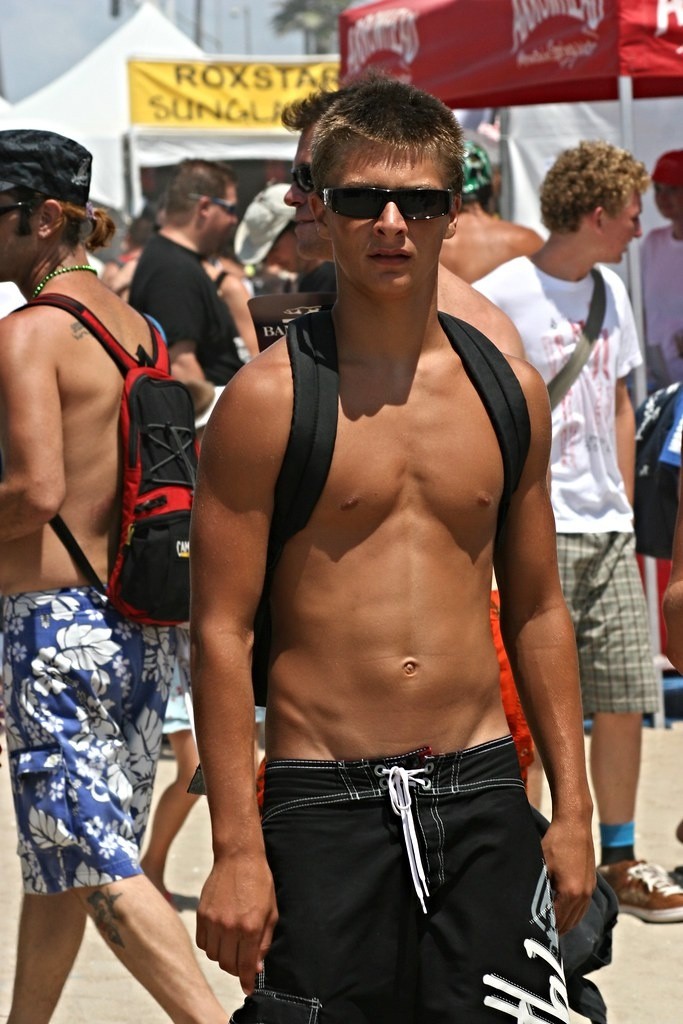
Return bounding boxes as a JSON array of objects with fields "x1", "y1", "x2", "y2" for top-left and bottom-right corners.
[
  {"x1": 313, "y1": 184, "x2": 462, "y2": 222},
  {"x1": 186, "y1": 192, "x2": 238, "y2": 215},
  {"x1": 291, "y1": 163, "x2": 314, "y2": 192}
]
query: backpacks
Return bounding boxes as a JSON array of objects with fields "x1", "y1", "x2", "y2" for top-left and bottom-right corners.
[
  {"x1": 10, "y1": 298, "x2": 199, "y2": 627},
  {"x1": 635, "y1": 383, "x2": 683, "y2": 558}
]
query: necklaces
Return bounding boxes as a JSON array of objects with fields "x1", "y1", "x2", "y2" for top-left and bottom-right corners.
[{"x1": 31, "y1": 265, "x2": 97, "y2": 296}]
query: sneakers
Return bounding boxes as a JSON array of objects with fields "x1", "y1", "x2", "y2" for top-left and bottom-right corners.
[{"x1": 596, "y1": 857, "x2": 683, "y2": 923}]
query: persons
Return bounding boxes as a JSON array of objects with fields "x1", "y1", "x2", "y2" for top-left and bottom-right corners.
[
  {"x1": 193, "y1": 78, "x2": 600, "y2": 1024},
  {"x1": 0, "y1": 88, "x2": 683, "y2": 1024}
]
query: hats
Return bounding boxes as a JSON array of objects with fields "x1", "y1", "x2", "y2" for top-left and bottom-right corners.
[
  {"x1": 0, "y1": 129, "x2": 92, "y2": 207},
  {"x1": 652, "y1": 150, "x2": 683, "y2": 188},
  {"x1": 456, "y1": 137, "x2": 492, "y2": 193},
  {"x1": 233, "y1": 182, "x2": 296, "y2": 266}
]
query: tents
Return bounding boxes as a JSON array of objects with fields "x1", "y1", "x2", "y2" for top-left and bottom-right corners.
[{"x1": 0, "y1": 0, "x2": 683, "y2": 726}]
